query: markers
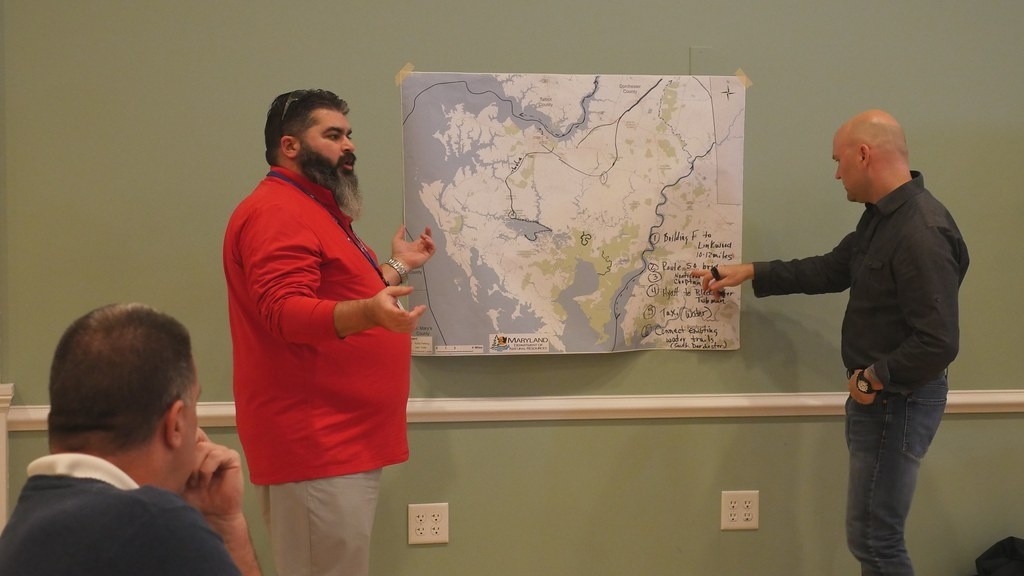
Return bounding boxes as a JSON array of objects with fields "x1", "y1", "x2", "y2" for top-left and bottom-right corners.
[{"x1": 711, "y1": 267, "x2": 725, "y2": 298}]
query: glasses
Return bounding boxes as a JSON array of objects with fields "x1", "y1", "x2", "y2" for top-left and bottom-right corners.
[{"x1": 282, "y1": 89, "x2": 308, "y2": 120}]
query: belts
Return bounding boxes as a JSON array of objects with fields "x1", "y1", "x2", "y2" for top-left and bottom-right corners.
[{"x1": 848, "y1": 367, "x2": 948, "y2": 382}]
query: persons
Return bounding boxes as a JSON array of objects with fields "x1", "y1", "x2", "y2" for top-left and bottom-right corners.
[
  {"x1": 221, "y1": 87, "x2": 437, "y2": 576},
  {"x1": 692, "y1": 108, "x2": 971, "y2": 576},
  {"x1": 0, "y1": 302, "x2": 263, "y2": 576}
]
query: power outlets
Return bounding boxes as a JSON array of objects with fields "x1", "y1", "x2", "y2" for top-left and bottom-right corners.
[
  {"x1": 407, "y1": 503, "x2": 450, "y2": 544},
  {"x1": 721, "y1": 491, "x2": 759, "y2": 531}
]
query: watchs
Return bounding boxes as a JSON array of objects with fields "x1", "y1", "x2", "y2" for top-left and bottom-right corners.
[{"x1": 855, "y1": 370, "x2": 876, "y2": 394}]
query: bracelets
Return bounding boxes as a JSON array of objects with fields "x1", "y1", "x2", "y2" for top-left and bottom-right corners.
[{"x1": 385, "y1": 257, "x2": 408, "y2": 283}]
